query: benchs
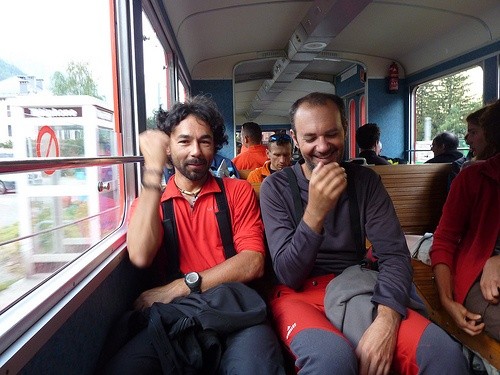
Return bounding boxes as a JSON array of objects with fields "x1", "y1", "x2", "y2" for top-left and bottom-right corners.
[
  {"x1": 410, "y1": 258, "x2": 500, "y2": 375},
  {"x1": 366, "y1": 163, "x2": 468, "y2": 235}
]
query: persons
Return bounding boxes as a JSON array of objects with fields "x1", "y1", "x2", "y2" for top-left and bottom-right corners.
[
  {"x1": 94, "y1": 94, "x2": 285, "y2": 375},
  {"x1": 162, "y1": 122, "x2": 392, "y2": 185},
  {"x1": 259, "y1": 92, "x2": 470, "y2": 374},
  {"x1": 424, "y1": 98, "x2": 500, "y2": 342}
]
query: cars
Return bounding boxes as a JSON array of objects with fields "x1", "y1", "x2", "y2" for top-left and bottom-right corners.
[{"x1": 0, "y1": 149, "x2": 43, "y2": 195}]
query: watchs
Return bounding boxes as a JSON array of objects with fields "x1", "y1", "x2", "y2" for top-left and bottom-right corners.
[{"x1": 184, "y1": 271, "x2": 201, "y2": 294}]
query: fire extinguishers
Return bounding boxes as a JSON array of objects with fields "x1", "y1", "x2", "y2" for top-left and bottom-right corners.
[{"x1": 389, "y1": 62, "x2": 399, "y2": 90}]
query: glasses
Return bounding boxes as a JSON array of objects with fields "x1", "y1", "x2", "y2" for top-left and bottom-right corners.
[{"x1": 269, "y1": 135, "x2": 293, "y2": 144}]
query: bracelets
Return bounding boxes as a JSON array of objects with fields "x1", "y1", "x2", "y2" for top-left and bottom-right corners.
[{"x1": 140, "y1": 166, "x2": 163, "y2": 191}]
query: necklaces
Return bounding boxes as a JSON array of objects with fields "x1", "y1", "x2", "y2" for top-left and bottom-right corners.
[{"x1": 176, "y1": 185, "x2": 201, "y2": 198}]
